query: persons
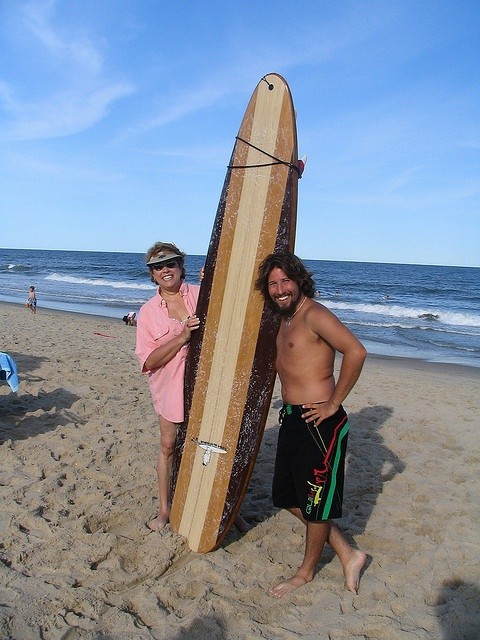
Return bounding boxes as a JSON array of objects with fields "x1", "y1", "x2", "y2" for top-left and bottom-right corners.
[
  {"x1": 27, "y1": 286, "x2": 37, "y2": 314},
  {"x1": 123, "y1": 312, "x2": 136, "y2": 326},
  {"x1": 199, "y1": 250, "x2": 368, "y2": 600},
  {"x1": 134, "y1": 241, "x2": 201, "y2": 533}
]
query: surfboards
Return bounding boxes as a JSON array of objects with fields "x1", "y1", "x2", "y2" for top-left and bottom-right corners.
[{"x1": 166, "y1": 72, "x2": 298, "y2": 553}]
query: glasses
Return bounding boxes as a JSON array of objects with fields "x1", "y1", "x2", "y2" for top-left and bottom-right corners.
[{"x1": 152, "y1": 262, "x2": 178, "y2": 271}]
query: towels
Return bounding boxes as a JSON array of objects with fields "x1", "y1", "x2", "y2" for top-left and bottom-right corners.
[{"x1": 0, "y1": 353, "x2": 20, "y2": 392}]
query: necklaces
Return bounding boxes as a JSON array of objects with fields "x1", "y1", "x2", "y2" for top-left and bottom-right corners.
[{"x1": 286, "y1": 296, "x2": 308, "y2": 327}]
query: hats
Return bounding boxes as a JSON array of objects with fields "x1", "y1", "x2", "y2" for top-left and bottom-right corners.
[{"x1": 146, "y1": 249, "x2": 183, "y2": 265}]
